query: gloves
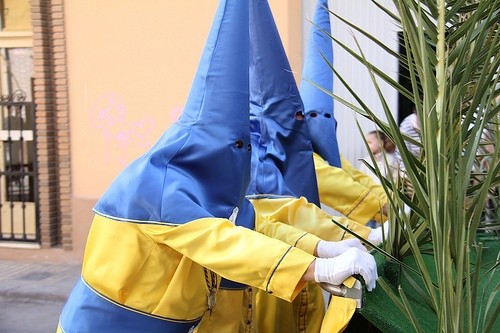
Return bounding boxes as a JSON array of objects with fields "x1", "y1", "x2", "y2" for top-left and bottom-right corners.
[
  {"x1": 368, "y1": 203, "x2": 411, "y2": 246},
  {"x1": 317, "y1": 237, "x2": 367, "y2": 258},
  {"x1": 315, "y1": 246, "x2": 378, "y2": 292}
]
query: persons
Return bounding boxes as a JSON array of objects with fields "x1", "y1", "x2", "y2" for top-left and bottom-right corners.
[
  {"x1": 57, "y1": 0, "x2": 378, "y2": 333},
  {"x1": 359, "y1": 90, "x2": 500, "y2": 212},
  {"x1": 298, "y1": 0, "x2": 412, "y2": 333},
  {"x1": 194, "y1": 0, "x2": 407, "y2": 333}
]
